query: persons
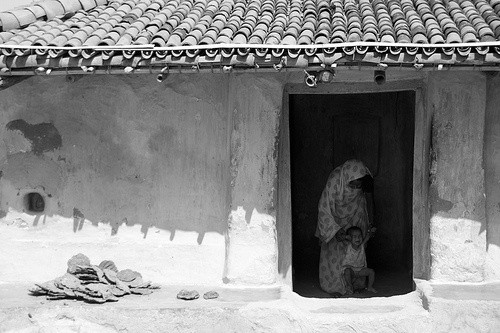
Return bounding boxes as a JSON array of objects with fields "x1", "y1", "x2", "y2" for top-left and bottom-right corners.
[
  {"x1": 336, "y1": 226, "x2": 376, "y2": 296},
  {"x1": 316, "y1": 160, "x2": 376, "y2": 295}
]
